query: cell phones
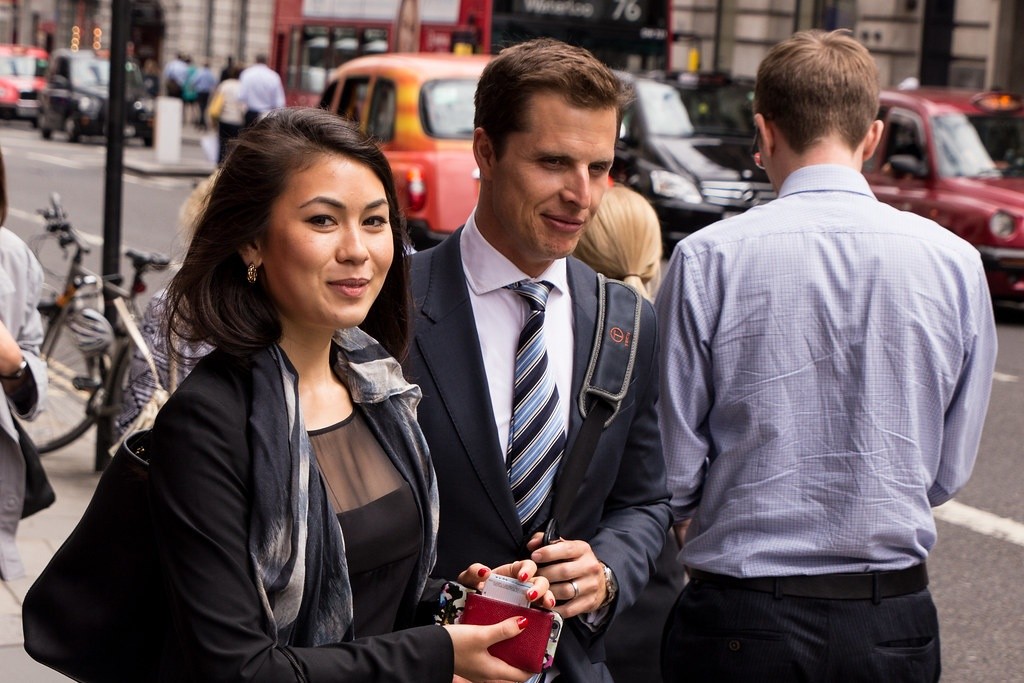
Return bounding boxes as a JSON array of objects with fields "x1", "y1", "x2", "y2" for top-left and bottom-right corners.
[{"x1": 429, "y1": 580, "x2": 562, "y2": 672}]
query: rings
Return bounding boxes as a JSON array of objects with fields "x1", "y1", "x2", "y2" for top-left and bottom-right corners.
[{"x1": 569, "y1": 581, "x2": 579, "y2": 599}]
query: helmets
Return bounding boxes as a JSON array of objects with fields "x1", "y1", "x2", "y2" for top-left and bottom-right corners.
[{"x1": 68, "y1": 309, "x2": 114, "y2": 354}]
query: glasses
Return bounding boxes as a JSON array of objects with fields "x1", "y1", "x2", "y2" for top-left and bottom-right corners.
[{"x1": 749, "y1": 122, "x2": 769, "y2": 171}]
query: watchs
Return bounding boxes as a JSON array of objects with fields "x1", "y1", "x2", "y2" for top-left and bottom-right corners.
[
  {"x1": 9, "y1": 357, "x2": 27, "y2": 379},
  {"x1": 598, "y1": 561, "x2": 618, "y2": 609}
]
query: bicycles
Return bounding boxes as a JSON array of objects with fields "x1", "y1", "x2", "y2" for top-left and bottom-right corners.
[{"x1": 9, "y1": 191, "x2": 186, "y2": 471}]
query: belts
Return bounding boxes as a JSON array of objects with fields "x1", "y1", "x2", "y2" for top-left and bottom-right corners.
[{"x1": 687, "y1": 562, "x2": 931, "y2": 599}]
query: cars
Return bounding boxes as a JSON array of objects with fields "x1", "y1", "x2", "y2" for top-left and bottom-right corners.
[
  {"x1": 604, "y1": 67, "x2": 780, "y2": 257},
  {"x1": 736, "y1": 77, "x2": 1024, "y2": 308},
  {"x1": 0, "y1": 43, "x2": 51, "y2": 129},
  {"x1": 318, "y1": 51, "x2": 500, "y2": 252},
  {"x1": 36, "y1": 47, "x2": 157, "y2": 148}
]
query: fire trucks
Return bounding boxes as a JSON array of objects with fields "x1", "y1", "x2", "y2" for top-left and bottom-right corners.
[{"x1": 266, "y1": 0, "x2": 493, "y2": 114}]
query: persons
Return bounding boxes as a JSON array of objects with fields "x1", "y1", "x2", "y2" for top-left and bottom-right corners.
[
  {"x1": 401, "y1": 28, "x2": 998, "y2": 683},
  {"x1": 0, "y1": 144, "x2": 50, "y2": 581},
  {"x1": 143, "y1": 50, "x2": 286, "y2": 170},
  {"x1": 150, "y1": 106, "x2": 555, "y2": 683}
]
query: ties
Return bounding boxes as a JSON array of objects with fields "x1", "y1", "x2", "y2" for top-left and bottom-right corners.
[{"x1": 501, "y1": 279, "x2": 566, "y2": 682}]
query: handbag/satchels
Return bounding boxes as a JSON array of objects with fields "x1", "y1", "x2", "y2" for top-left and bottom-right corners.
[
  {"x1": 208, "y1": 89, "x2": 225, "y2": 126},
  {"x1": 185, "y1": 64, "x2": 199, "y2": 99},
  {"x1": 24, "y1": 429, "x2": 211, "y2": 683},
  {"x1": 109, "y1": 296, "x2": 171, "y2": 456},
  {"x1": 10, "y1": 406, "x2": 55, "y2": 518},
  {"x1": 167, "y1": 75, "x2": 181, "y2": 96}
]
